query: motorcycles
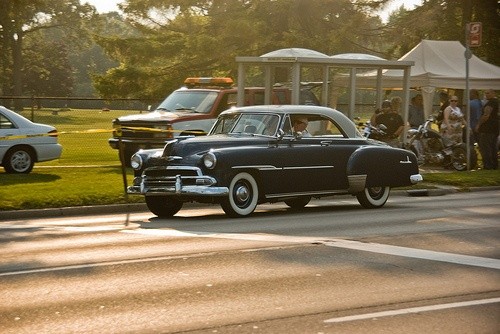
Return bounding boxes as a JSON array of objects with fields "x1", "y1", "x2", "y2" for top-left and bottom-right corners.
[
  {"x1": 401, "y1": 118, "x2": 477, "y2": 171},
  {"x1": 358, "y1": 120, "x2": 389, "y2": 138}
]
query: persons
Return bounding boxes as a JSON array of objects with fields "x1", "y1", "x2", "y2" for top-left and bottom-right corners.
[
  {"x1": 287, "y1": 115, "x2": 313, "y2": 137},
  {"x1": 372, "y1": 88, "x2": 500, "y2": 170}
]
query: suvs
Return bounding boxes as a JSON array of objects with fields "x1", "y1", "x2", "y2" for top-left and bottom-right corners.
[{"x1": 109, "y1": 75, "x2": 292, "y2": 169}]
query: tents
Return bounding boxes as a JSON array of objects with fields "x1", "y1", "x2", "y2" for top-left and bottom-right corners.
[{"x1": 328, "y1": 39, "x2": 500, "y2": 131}]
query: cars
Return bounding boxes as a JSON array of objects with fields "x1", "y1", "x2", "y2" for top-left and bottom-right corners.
[
  {"x1": 124, "y1": 103, "x2": 423, "y2": 218},
  {"x1": 0, "y1": 105, "x2": 62, "y2": 174}
]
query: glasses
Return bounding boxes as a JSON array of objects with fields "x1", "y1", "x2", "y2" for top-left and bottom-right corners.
[
  {"x1": 450, "y1": 100, "x2": 458, "y2": 102},
  {"x1": 294, "y1": 120, "x2": 304, "y2": 124}
]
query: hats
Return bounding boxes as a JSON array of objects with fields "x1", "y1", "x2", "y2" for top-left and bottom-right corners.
[
  {"x1": 448, "y1": 95, "x2": 460, "y2": 104},
  {"x1": 381, "y1": 100, "x2": 393, "y2": 108}
]
